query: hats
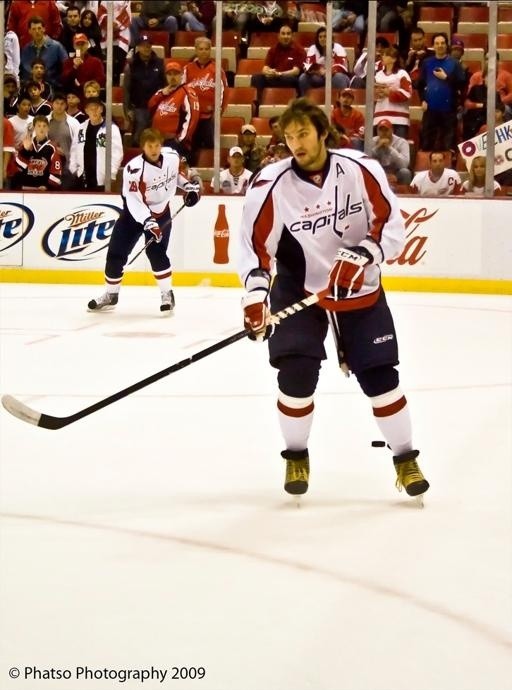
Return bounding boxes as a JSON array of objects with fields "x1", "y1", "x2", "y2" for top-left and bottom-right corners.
[
  {"x1": 77, "y1": 97, "x2": 106, "y2": 117},
  {"x1": 164, "y1": 62, "x2": 181, "y2": 72},
  {"x1": 341, "y1": 88, "x2": 354, "y2": 97},
  {"x1": 450, "y1": 40, "x2": 465, "y2": 49},
  {"x1": 242, "y1": 125, "x2": 256, "y2": 134},
  {"x1": 378, "y1": 120, "x2": 392, "y2": 130},
  {"x1": 73, "y1": 33, "x2": 88, "y2": 45},
  {"x1": 230, "y1": 146, "x2": 243, "y2": 157},
  {"x1": 135, "y1": 35, "x2": 150, "y2": 45}
]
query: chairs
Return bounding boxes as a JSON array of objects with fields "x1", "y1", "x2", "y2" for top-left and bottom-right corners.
[{"x1": 101, "y1": 2, "x2": 512, "y2": 197}]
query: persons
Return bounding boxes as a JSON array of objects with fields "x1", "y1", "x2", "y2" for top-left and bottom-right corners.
[
  {"x1": 89, "y1": 127, "x2": 201, "y2": 316},
  {"x1": 0, "y1": 1, "x2": 512, "y2": 198},
  {"x1": 241, "y1": 97, "x2": 429, "y2": 497}
]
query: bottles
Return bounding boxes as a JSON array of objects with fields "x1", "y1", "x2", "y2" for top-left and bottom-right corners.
[{"x1": 212, "y1": 203, "x2": 231, "y2": 266}]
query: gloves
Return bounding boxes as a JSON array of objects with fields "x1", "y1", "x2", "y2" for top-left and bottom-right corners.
[
  {"x1": 327, "y1": 240, "x2": 384, "y2": 299},
  {"x1": 243, "y1": 269, "x2": 276, "y2": 340},
  {"x1": 184, "y1": 183, "x2": 201, "y2": 206},
  {"x1": 144, "y1": 217, "x2": 163, "y2": 243}
]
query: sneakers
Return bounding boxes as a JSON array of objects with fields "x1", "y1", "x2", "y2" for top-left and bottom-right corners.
[
  {"x1": 88, "y1": 293, "x2": 118, "y2": 310},
  {"x1": 392, "y1": 450, "x2": 429, "y2": 496},
  {"x1": 160, "y1": 290, "x2": 174, "y2": 310},
  {"x1": 281, "y1": 449, "x2": 309, "y2": 495}
]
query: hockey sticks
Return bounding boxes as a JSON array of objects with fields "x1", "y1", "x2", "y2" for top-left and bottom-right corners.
[{"x1": 1, "y1": 290, "x2": 332, "y2": 430}]
